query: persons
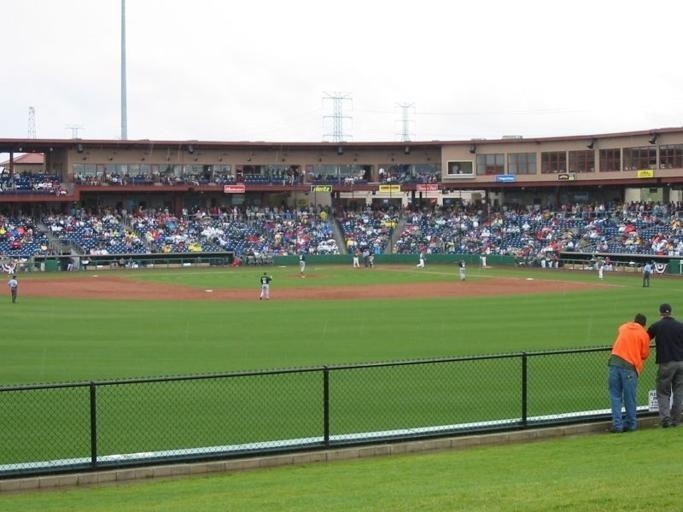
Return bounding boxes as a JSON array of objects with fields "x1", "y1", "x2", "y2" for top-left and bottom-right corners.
[
  {"x1": 594, "y1": 258, "x2": 605, "y2": 280},
  {"x1": 459, "y1": 258, "x2": 468, "y2": 281},
  {"x1": 1, "y1": 165, "x2": 683, "y2": 274},
  {"x1": 646, "y1": 304, "x2": 683, "y2": 427},
  {"x1": 9, "y1": 275, "x2": 18, "y2": 303},
  {"x1": 607, "y1": 313, "x2": 650, "y2": 432},
  {"x1": 640, "y1": 261, "x2": 655, "y2": 288},
  {"x1": 259, "y1": 272, "x2": 273, "y2": 301}
]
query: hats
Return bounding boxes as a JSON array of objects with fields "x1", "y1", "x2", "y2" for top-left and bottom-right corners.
[
  {"x1": 659, "y1": 303, "x2": 672, "y2": 317},
  {"x1": 635, "y1": 315, "x2": 646, "y2": 325}
]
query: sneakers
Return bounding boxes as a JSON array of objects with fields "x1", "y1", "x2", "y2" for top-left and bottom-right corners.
[
  {"x1": 628, "y1": 427, "x2": 637, "y2": 430},
  {"x1": 662, "y1": 419, "x2": 679, "y2": 428},
  {"x1": 611, "y1": 429, "x2": 623, "y2": 433}
]
